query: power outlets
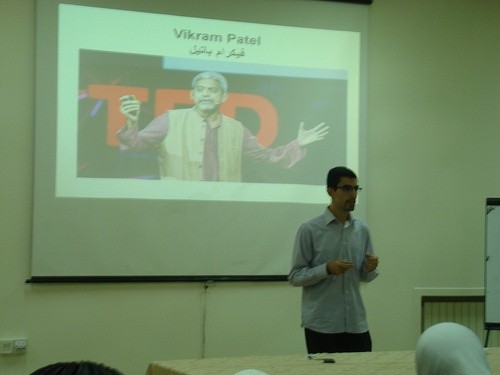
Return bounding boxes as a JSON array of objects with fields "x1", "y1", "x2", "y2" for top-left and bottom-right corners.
[{"x1": 0, "y1": 339, "x2": 28, "y2": 354}]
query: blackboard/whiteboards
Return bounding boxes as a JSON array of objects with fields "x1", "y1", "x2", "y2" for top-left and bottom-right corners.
[{"x1": 484, "y1": 197, "x2": 500, "y2": 330}]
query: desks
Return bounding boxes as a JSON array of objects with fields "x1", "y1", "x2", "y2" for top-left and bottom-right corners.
[{"x1": 147, "y1": 347, "x2": 500, "y2": 375}]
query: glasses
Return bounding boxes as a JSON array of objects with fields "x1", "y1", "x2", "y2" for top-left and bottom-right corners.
[{"x1": 337, "y1": 184, "x2": 359, "y2": 192}]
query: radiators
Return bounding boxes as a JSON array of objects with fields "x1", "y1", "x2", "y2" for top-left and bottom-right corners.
[{"x1": 420, "y1": 296, "x2": 500, "y2": 348}]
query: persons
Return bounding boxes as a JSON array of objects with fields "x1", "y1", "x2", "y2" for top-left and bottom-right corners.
[
  {"x1": 288, "y1": 166, "x2": 380, "y2": 354},
  {"x1": 115, "y1": 71, "x2": 329, "y2": 183}
]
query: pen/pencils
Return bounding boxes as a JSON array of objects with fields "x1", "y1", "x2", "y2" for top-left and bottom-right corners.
[{"x1": 313, "y1": 357, "x2": 326, "y2": 359}]
